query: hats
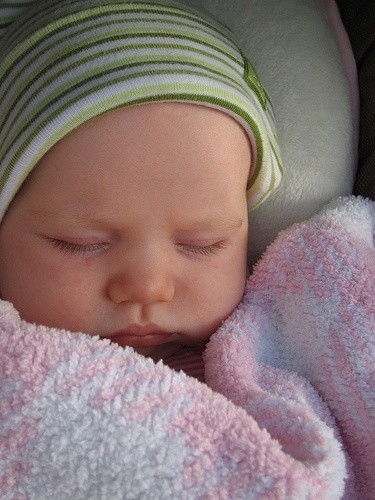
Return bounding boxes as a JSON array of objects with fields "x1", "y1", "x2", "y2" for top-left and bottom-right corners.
[{"x1": 0, "y1": 0, "x2": 284, "y2": 225}]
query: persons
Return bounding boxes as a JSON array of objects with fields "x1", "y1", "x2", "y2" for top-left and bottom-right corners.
[{"x1": 2, "y1": 0, "x2": 375, "y2": 500}]
